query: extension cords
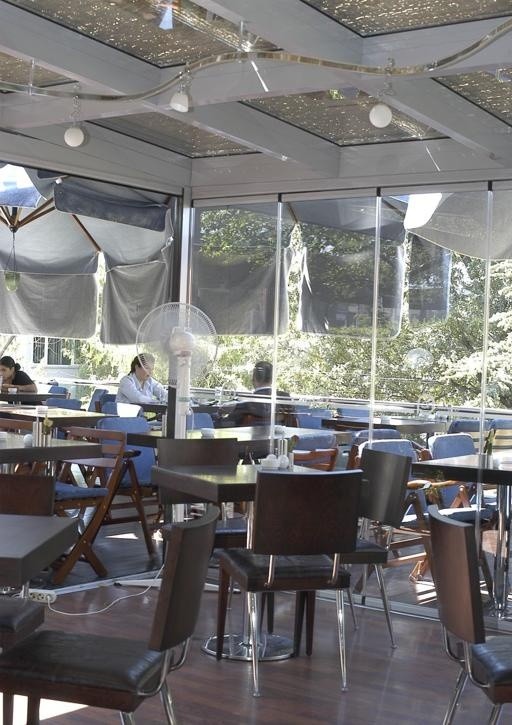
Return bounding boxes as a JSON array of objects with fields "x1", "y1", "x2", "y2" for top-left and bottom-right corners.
[{"x1": 21, "y1": 589, "x2": 56, "y2": 603}]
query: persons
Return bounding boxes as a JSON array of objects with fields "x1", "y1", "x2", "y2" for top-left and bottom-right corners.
[
  {"x1": 219, "y1": 362, "x2": 297, "y2": 427},
  {"x1": 115, "y1": 353, "x2": 169, "y2": 406},
  {"x1": 0, "y1": 356, "x2": 37, "y2": 393}
]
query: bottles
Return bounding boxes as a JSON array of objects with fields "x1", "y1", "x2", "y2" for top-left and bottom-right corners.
[
  {"x1": 279, "y1": 440, "x2": 288, "y2": 468},
  {"x1": 36, "y1": 405, "x2": 47, "y2": 446}
]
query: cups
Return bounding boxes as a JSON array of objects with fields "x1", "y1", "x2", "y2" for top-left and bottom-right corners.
[
  {"x1": 214, "y1": 387, "x2": 222, "y2": 405},
  {"x1": 224, "y1": 391, "x2": 233, "y2": 401},
  {"x1": 232, "y1": 389, "x2": 236, "y2": 399}
]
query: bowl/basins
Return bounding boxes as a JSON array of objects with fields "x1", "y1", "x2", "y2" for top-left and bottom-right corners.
[
  {"x1": 0, "y1": 431, "x2": 8, "y2": 439},
  {"x1": 200, "y1": 428, "x2": 215, "y2": 438},
  {"x1": 260, "y1": 458, "x2": 279, "y2": 468},
  {"x1": 8, "y1": 387, "x2": 17, "y2": 393}
]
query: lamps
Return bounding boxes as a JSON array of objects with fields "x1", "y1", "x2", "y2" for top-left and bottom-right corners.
[
  {"x1": 169, "y1": 67, "x2": 189, "y2": 112},
  {"x1": 369, "y1": 59, "x2": 393, "y2": 129},
  {"x1": 63, "y1": 84, "x2": 85, "y2": 148},
  {"x1": 136, "y1": 302, "x2": 219, "y2": 522}
]
